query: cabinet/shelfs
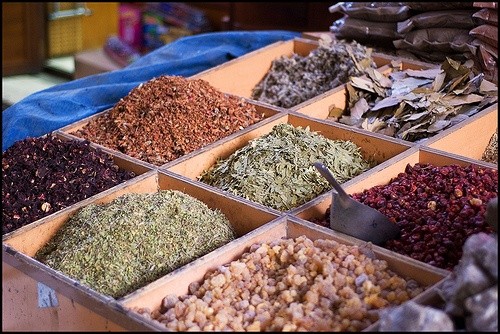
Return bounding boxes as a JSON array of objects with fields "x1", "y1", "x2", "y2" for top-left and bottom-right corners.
[{"x1": 2, "y1": 31, "x2": 500, "y2": 331}]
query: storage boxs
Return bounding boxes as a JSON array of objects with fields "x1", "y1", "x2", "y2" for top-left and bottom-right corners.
[{"x1": 74, "y1": 49, "x2": 120, "y2": 80}]
